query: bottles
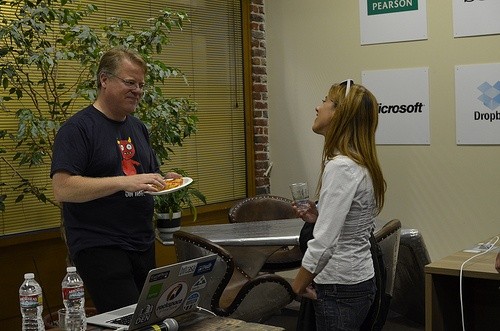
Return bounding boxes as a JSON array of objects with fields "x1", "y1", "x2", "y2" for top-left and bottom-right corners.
[
  {"x1": 18, "y1": 271, "x2": 45, "y2": 331},
  {"x1": 60, "y1": 266, "x2": 87, "y2": 330}
]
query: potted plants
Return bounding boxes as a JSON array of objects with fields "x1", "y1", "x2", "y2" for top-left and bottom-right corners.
[{"x1": 154, "y1": 168, "x2": 206, "y2": 240}]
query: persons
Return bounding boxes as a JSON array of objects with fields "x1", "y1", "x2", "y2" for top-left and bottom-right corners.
[
  {"x1": 291, "y1": 79, "x2": 387, "y2": 331},
  {"x1": 50, "y1": 48, "x2": 182, "y2": 314}
]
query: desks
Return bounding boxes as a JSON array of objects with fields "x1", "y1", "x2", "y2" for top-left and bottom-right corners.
[
  {"x1": 47, "y1": 312, "x2": 286, "y2": 331},
  {"x1": 424, "y1": 246, "x2": 500, "y2": 331},
  {"x1": 180, "y1": 218, "x2": 306, "y2": 278}
]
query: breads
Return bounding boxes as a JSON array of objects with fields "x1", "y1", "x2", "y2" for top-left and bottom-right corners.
[{"x1": 152, "y1": 177, "x2": 184, "y2": 192}]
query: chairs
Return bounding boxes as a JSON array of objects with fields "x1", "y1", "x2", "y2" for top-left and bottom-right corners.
[
  {"x1": 229, "y1": 194, "x2": 300, "y2": 223},
  {"x1": 401, "y1": 229, "x2": 432, "y2": 303},
  {"x1": 174, "y1": 230, "x2": 296, "y2": 321},
  {"x1": 374, "y1": 219, "x2": 401, "y2": 326}
]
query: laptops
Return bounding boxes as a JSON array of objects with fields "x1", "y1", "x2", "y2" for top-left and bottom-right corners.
[{"x1": 86, "y1": 254, "x2": 217, "y2": 331}]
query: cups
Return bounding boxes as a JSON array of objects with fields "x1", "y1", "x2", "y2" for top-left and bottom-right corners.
[
  {"x1": 288, "y1": 181, "x2": 310, "y2": 210},
  {"x1": 140, "y1": 176, "x2": 195, "y2": 197}
]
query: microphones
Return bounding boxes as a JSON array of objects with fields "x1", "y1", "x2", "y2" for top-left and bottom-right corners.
[{"x1": 137, "y1": 318, "x2": 179, "y2": 331}]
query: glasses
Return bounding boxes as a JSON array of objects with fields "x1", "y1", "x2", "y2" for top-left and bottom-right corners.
[
  {"x1": 339, "y1": 79, "x2": 354, "y2": 97},
  {"x1": 104, "y1": 71, "x2": 148, "y2": 93}
]
